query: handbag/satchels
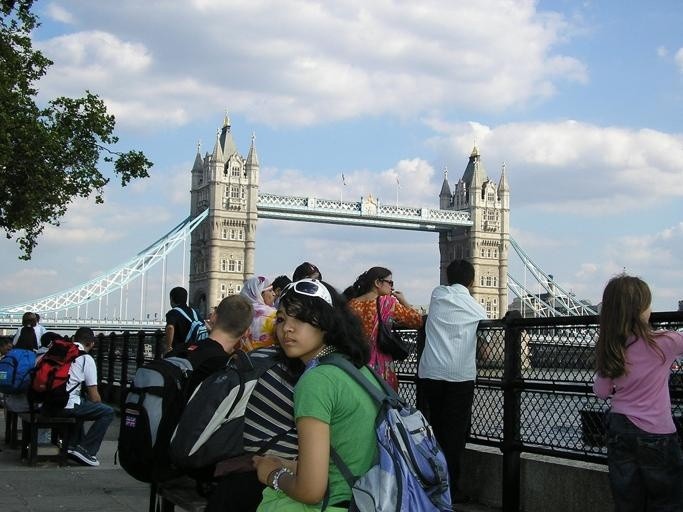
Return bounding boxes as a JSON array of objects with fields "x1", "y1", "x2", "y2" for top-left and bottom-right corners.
[
  {"x1": 376, "y1": 294, "x2": 408, "y2": 361},
  {"x1": 198, "y1": 453, "x2": 267, "y2": 511}
]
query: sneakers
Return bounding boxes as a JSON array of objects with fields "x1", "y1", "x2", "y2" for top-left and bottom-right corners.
[
  {"x1": 72, "y1": 445, "x2": 100, "y2": 466},
  {"x1": 67, "y1": 446, "x2": 77, "y2": 454}
]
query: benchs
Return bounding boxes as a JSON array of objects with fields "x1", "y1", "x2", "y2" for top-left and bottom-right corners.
[
  {"x1": 3, "y1": 406, "x2": 80, "y2": 468},
  {"x1": 149, "y1": 481, "x2": 206, "y2": 512}
]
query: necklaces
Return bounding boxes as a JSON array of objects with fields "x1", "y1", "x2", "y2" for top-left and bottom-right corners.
[{"x1": 309, "y1": 346, "x2": 337, "y2": 368}]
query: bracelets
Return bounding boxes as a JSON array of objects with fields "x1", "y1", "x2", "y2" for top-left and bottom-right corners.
[{"x1": 272, "y1": 468, "x2": 293, "y2": 494}]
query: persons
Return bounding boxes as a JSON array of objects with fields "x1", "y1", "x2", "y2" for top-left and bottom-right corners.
[
  {"x1": 235, "y1": 277, "x2": 279, "y2": 354},
  {"x1": 166, "y1": 287, "x2": 212, "y2": 354},
  {"x1": 252, "y1": 277, "x2": 387, "y2": 512},
  {"x1": 417, "y1": 258, "x2": 489, "y2": 502},
  {"x1": 201, "y1": 345, "x2": 306, "y2": 512},
  {"x1": 344, "y1": 267, "x2": 423, "y2": 396},
  {"x1": 0, "y1": 327, "x2": 44, "y2": 423},
  {"x1": 595, "y1": 276, "x2": 682, "y2": 512},
  {"x1": 272, "y1": 276, "x2": 299, "y2": 297},
  {"x1": 183, "y1": 295, "x2": 256, "y2": 397},
  {"x1": 36, "y1": 327, "x2": 115, "y2": 466},
  {"x1": 27, "y1": 331, "x2": 62, "y2": 369},
  {"x1": 12, "y1": 312, "x2": 43, "y2": 349}
]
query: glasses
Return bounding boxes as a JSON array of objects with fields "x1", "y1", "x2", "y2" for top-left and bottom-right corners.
[
  {"x1": 381, "y1": 279, "x2": 392, "y2": 285},
  {"x1": 274, "y1": 279, "x2": 334, "y2": 308}
]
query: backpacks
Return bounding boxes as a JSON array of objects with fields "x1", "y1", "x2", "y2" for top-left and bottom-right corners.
[
  {"x1": 311, "y1": 353, "x2": 453, "y2": 512},
  {"x1": 171, "y1": 306, "x2": 210, "y2": 345},
  {"x1": 27, "y1": 340, "x2": 90, "y2": 411},
  {"x1": 161, "y1": 345, "x2": 300, "y2": 474},
  {"x1": 116, "y1": 344, "x2": 229, "y2": 483},
  {"x1": 0, "y1": 345, "x2": 50, "y2": 393}
]
